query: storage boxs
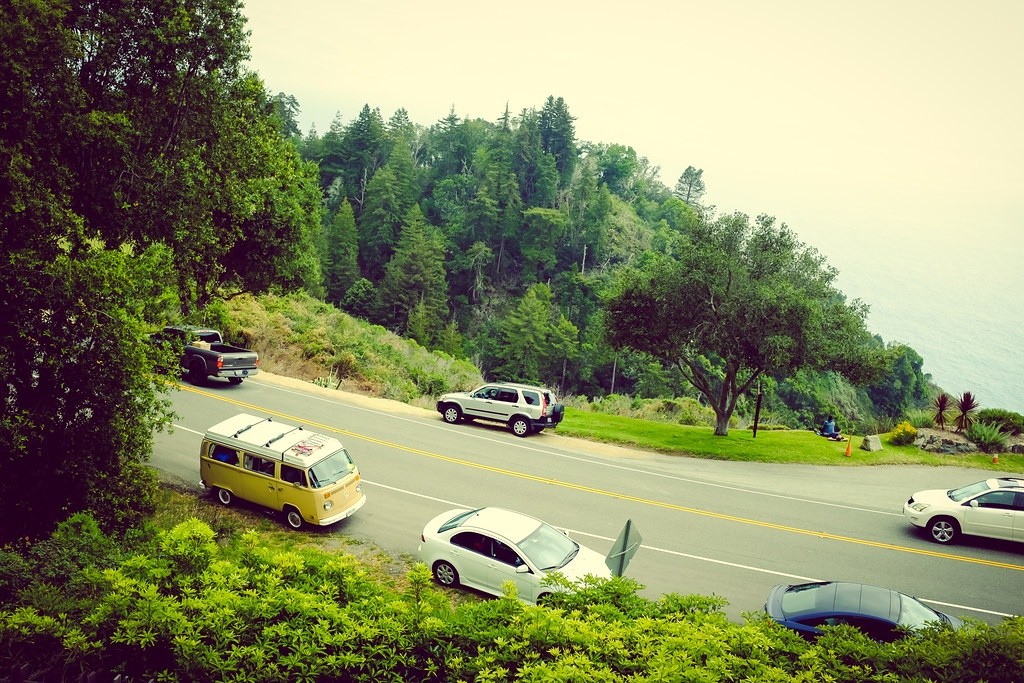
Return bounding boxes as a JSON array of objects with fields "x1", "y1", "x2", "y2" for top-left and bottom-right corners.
[{"x1": 192, "y1": 341, "x2": 211, "y2": 350}]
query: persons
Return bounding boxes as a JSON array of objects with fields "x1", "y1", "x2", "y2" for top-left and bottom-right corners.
[{"x1": 819, "y1": 416, "x2": 835, "y2": 435}]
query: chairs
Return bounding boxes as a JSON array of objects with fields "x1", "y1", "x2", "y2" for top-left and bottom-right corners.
[
  {"x1": 285, "y1": 469, "x2": 302, "y2": 484},
  {"x1": 252, "y1": 458, "x2": 263, "y2": 471}
]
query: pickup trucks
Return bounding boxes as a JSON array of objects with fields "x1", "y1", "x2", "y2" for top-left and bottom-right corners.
[{"x1": 147, "y1": 326, "x2": 260, "y2": 387}]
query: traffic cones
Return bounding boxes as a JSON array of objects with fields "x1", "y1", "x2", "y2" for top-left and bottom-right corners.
[
  {"x1": 992, "y1": 451, "x2": 1000, "y2": 465},
  {"x1": 843, "y1": 432, "x2": 852, "y2": 458}
]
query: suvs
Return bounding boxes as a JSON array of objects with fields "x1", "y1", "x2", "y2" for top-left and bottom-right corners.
[{"x1": 436, "y1": 382, "x2": 566, "y2": 439}]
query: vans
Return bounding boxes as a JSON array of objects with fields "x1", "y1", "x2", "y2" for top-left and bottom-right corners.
[{"x1": 198, "y1": 414, "x2": 367, "y2": 532}]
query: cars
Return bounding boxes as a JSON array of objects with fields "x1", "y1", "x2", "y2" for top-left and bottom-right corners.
[
  {"x1": 417, "y1": 506, "x2": 614, "y2": 613},
  {"x1": 760, "y1": 580, "x2": 975, "y2": 649},
  {"x1": 903, "y1": 475, "x2": 1024, "y2": 545}
]
